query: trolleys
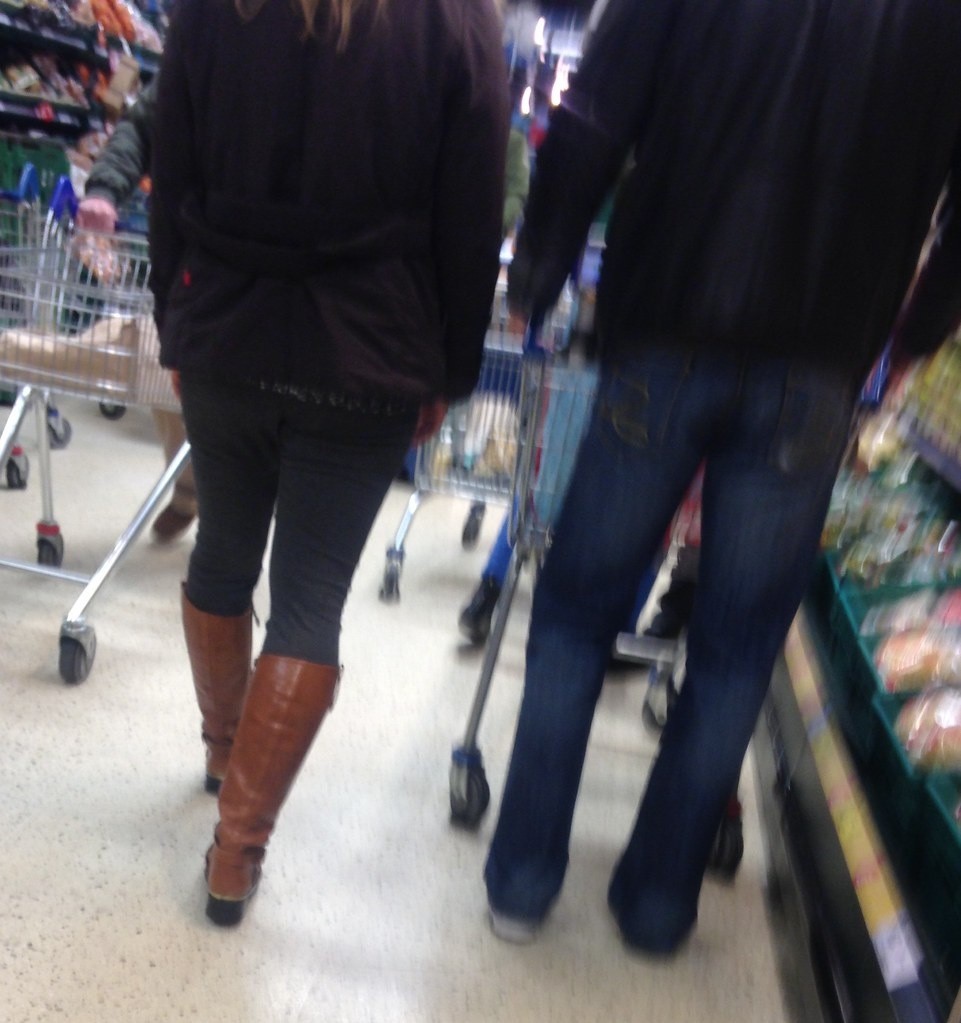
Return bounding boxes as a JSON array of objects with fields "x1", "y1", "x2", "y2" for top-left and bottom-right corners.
[
  {"x1": 376, "y1": 239, "x2": 573, "y2": 601},
  {"x1": 447, "y1": 241, "x2": 890, "y2": 872},
  {"x1": 1, "y1": 162, "x2": 72, "y2": 491},
  {"x1": 66, "y1": 177, "x2": 152, "y2": 419},
  {"x1": 0, "y1": 173, "x2": 194, "y2": 684}
]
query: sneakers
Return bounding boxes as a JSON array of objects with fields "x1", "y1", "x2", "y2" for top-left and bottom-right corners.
[
  {"x1": 484, "y1": 892, "x2": 540, "y2": 946},
  {"x1": 616, "y1": 920, "x2": 686, "y2": 968},
  {"x1": 150, "y1": 504, "x2": 195, "y2": 541}
]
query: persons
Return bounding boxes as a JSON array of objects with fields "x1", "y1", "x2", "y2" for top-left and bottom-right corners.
[
  {"x1": 502, "y1": 125, "x2": 530, "y2": 236},
  {"x1": 140, "y1": 0, "x2": 514, "y2": 930},
  {"x1": 606, "y1": 522, "x2": 709, "y2": 665},
  {"x1": 479, "y1": 0, "x2": 961, "y2": 961},
  {"x1": 456, "y1": 487, "x2": 673, "y2": 672},
  {"x1": 74, "y1": 71, "x2": 200, "y2": 539}
]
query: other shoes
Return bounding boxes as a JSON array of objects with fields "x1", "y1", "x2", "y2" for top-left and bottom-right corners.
[
  {"x1": 456, "y1": 577, "x2": 502, "y2": 647},
  {"x1": 607, "y1": 657, "x2": 647, "y2": 673}
]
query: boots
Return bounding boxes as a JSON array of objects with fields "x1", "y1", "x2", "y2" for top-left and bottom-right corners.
[
  {"x1": 179, "y1": 581, "x2": 255, "y2": 795},
  {"x1": 204, "y1": 655, "x2": 338, "y2": 930}
]
